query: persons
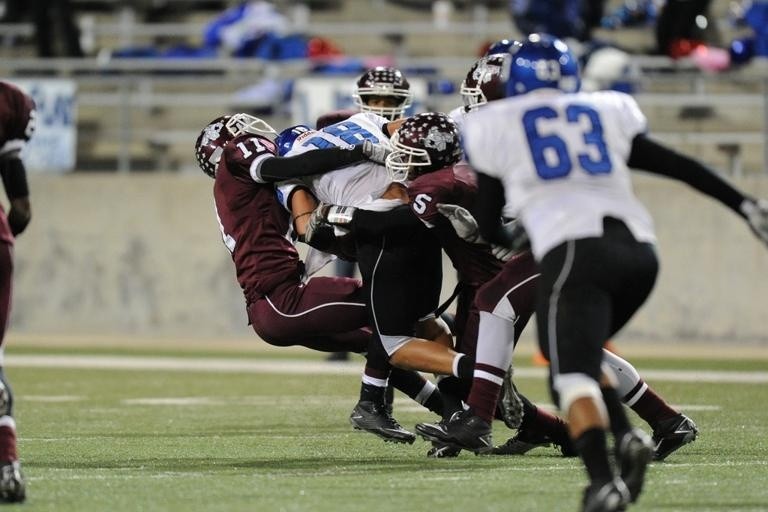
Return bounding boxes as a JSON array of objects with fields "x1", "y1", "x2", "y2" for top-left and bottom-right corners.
[
  {"x1": 194, "y1": 32, "x2": 768, "y2": 512},
  {"x1": 193, "y1": 1, "x2": 369, "y2": 99},
  {"x1": 514, "y1": 0, "x2": 767, "y2": 75},
  {"x1": 1, "y1": 82, "x2": 35, "y2": 504}
]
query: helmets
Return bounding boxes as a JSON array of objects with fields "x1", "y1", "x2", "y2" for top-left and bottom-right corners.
[
  {"x1": 271, "y1": 124, "x2": 310, "y2": 157},
  {"x1": 387, "y1": 110, "x2": 465, "y2": 182},
  {"x1": 193, "y1": 111, "x2": 283, "y2": 180},
  {"x1": 460, "y1": 32, "x2": 582, "y2": 109},
  {"x1": 353, "y1": 65, "x2": 412, "y2": 122}
]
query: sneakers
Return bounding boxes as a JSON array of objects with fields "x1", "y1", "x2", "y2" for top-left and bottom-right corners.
[
  {"x1": 0, "y1": 465, "x2": 26, "y2": 504},
  {"x1": 583, "y1": 476, "x2": 631, "y2": 512},
  {"x1": 416, "y1": 362, "x2": 550, "y2": 458},
  {"x1": 349, "y1": 397, "x2": 417, "y2": 446},
  {"x1": 621, "y1": 428, "x2": 655, "y2": 502},
  {"x1": 650, "y1": 415, "x2": 700, "y2": 461}
]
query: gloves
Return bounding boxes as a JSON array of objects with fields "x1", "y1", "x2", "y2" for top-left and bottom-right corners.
[
  {"x1": 434, "y1": 200, "x2": 479, "y2": 245},
  {"x1": 302, "y1": 201, "x2": 327, "y2": 244},
  {"x1": 361, "y1": 132, "x2": 390, "y2": 164}
]
